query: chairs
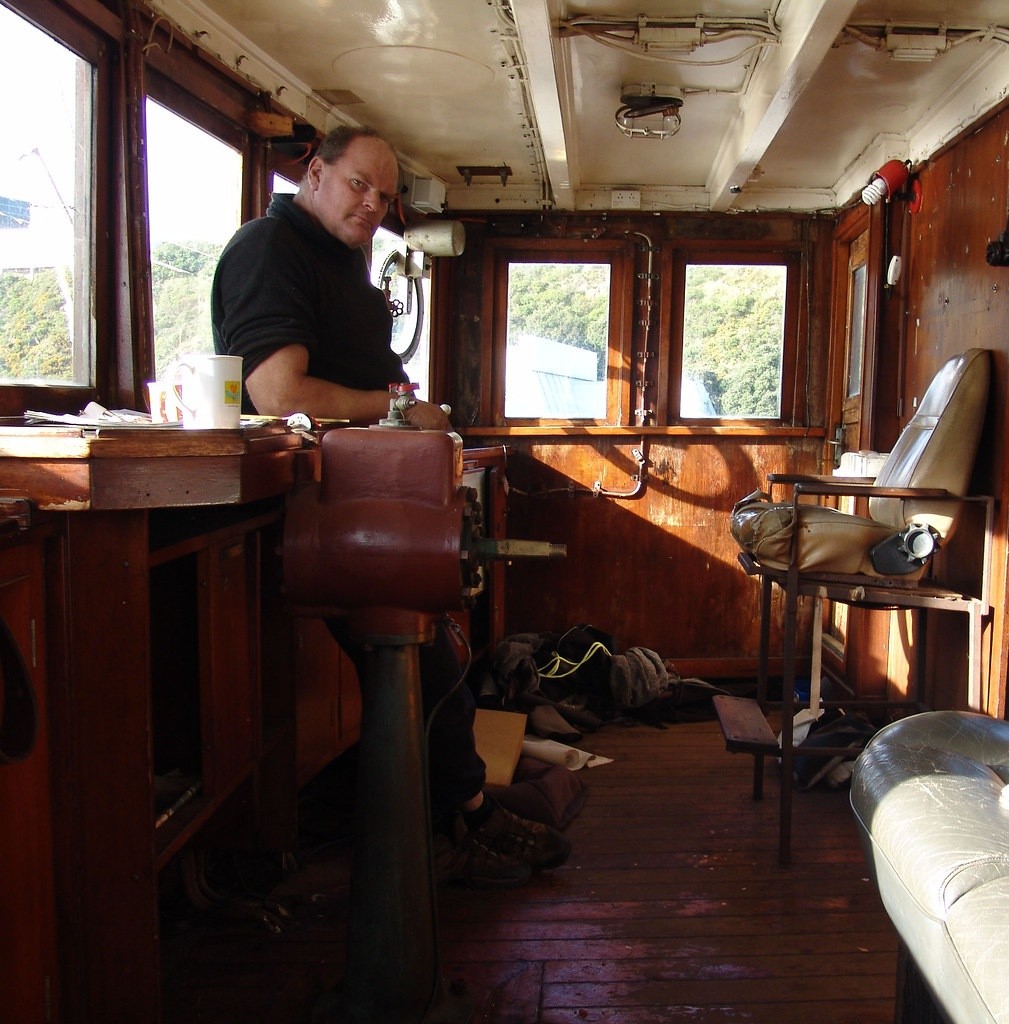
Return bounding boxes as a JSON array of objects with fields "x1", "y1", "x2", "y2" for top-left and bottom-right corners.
[{"x1": 712, "y1": 349, "x2": 996, "y2": 870}]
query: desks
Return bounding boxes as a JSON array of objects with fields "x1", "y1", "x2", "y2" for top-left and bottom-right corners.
[{"x1": 1, "y1": 416, "x2": 342, "y2": 1024}]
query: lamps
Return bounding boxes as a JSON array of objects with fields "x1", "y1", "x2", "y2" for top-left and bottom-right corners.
[
  {"x1": 613, "y1": 96, "x2": 683, "y2": 142},
  {"x1": 861, "y1": 159, "x2": 913, "y2": 206}
]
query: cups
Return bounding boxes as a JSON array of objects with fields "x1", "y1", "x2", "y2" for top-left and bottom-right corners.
[
  {"x1": 165, "y1": 354, "x2": 243, "y2": 429},
  {"x1": 146, "y1": 381, "x2": 182, "y2": 422}
]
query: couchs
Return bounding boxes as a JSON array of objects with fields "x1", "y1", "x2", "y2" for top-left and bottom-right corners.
[{"x1": 846, "y1": 711, "x2": 1009, "y2": 1024}]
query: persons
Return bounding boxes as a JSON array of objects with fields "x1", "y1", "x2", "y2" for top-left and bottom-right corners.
[{"x1": 210, "y1": 125, "x2": 570, "y2": 880}]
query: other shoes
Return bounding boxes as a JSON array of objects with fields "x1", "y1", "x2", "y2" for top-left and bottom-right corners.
[
  {"x1": 464, "y1": 800, "x2": 574, "y2": 872},
  {"x1": 431, "y1": 812, "x2": 534, "y2": 892}
]
